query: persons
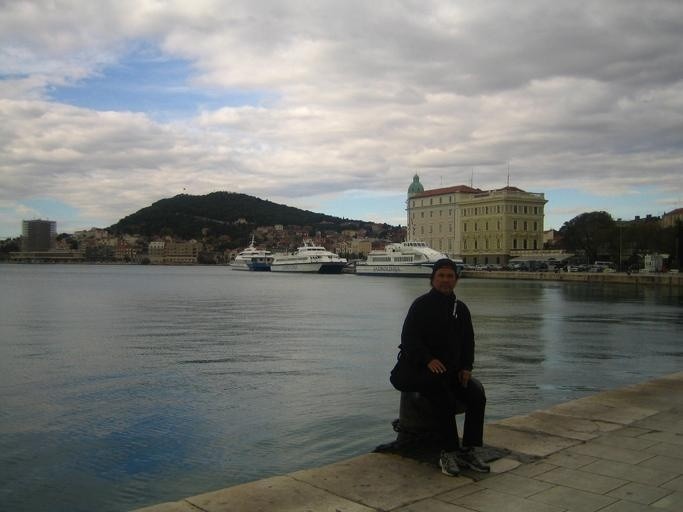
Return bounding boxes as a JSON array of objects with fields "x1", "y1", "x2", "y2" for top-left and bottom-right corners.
[{"x1": 388, "y1": 260, "x2": 491, "y2": 476}]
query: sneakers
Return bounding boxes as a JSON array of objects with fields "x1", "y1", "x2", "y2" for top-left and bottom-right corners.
[
  {"x1": 456, "y1": 448, "x2": 490, "y2": 474},
  {"x1": 438, "y1": 448, "x2": 462, "y2": 477}
]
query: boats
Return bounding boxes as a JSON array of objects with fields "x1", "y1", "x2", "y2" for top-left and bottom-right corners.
[
  {"x1": 229, "y1": 234, "x2": 273, "y2": 271},
  {"x1": 268, "y1": 244, "x2": 348, "y2": 273},
  {"x1": 354, "y1": 238, "x2": 448, "y2": 277}
]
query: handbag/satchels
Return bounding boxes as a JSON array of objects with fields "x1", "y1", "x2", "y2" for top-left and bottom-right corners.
[{"x1": 390, "y1": 350, "x2": 424, "y2": 392}]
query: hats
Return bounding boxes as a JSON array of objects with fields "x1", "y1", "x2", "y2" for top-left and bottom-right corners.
[{"x1": 432, "y1": 258, "x2": 457, "y2": 272}]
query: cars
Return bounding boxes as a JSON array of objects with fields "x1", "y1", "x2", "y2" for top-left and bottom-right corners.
[{"x1": 453, "y1": 258, "x2": 616, "y2": 274}]
